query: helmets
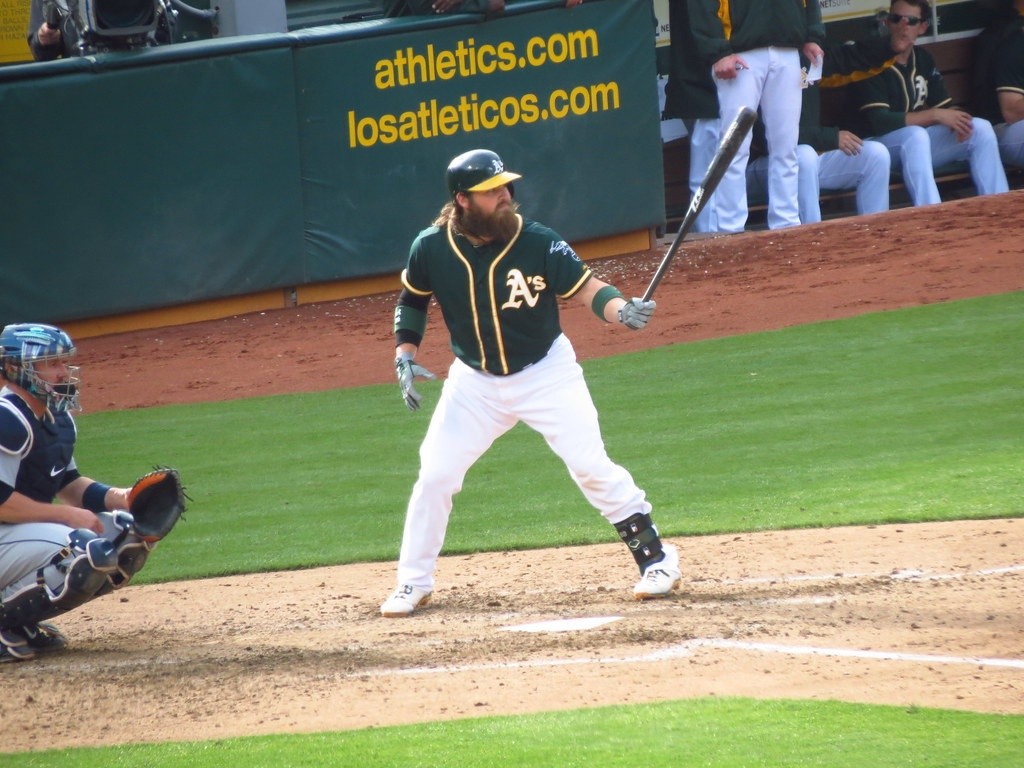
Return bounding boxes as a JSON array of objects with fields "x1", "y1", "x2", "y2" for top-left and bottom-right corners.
[
  {"x1": 446, "y1": 149, "x2": 522, "y2": 196},
  {"x1": 0, "y1": 322, "x2": 82, "y2": 415}
]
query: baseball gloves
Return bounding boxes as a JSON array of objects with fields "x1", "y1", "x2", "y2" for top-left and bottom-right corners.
[{"x1": 128, "y1": 467, "x2": 186, "y2": 544}]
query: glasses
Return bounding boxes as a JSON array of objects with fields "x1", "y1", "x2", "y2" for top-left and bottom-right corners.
[{"x1": 888, "y1": 13, "x2": 922, "y2": 26}]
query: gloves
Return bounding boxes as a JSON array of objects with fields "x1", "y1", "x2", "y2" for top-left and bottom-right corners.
[
  {"x1": 618, "y1": 296, "x2": 656, "y2": 331},
  {"x1": 394, "y1": 351, "x2": 436, "y2": 411}
]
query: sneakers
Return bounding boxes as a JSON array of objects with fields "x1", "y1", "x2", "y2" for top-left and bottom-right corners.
[
  {"x1": 633, "y1": 544, "x2": 681, "y2": 595},
  {"x1": 381, "y1": 584, "x2": 433, "y2": 617}
]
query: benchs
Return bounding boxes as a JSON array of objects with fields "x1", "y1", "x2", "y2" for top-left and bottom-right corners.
[{"x1": 664, "y1": 36, "x2": 1018, "y2": 226}]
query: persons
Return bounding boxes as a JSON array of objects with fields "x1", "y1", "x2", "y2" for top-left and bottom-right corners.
[
  {"x1": 973, "y1": 0, "x2": 1024, "y2": 170},
  {"x1": 746, "y1": 48, "x2": 890, "y2": 225},
  {"x1": 664, "y1": 0, "x2": 826, "y2": 235},
  {"x1": 0, "y1": 324, "x2": 157, "y2": 664},
  {"x1": 384, "y1": 0, "x2": 506, "y2": 18},
  {"x1": 832, "y1": 0, "x2": 1010, "y2": 206},
  {"x1": 27, "y1": 0, "x2": 171, "y2": 62},
  {"x1": 378, "y1": 148, "x2": 681, "y2": 619}
]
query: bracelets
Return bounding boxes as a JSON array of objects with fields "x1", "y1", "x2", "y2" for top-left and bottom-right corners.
[
  {"x1": 592, "y1": 285, "x2": 624, "y2": 323},
  {"x1": 82, "y1": 482, "x2": 112, "y2": 513}
]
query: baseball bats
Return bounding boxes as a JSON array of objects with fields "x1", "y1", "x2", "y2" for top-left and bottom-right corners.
[{"x1": 624, "y1": 105, "x2": 757, "y2": 331}]
